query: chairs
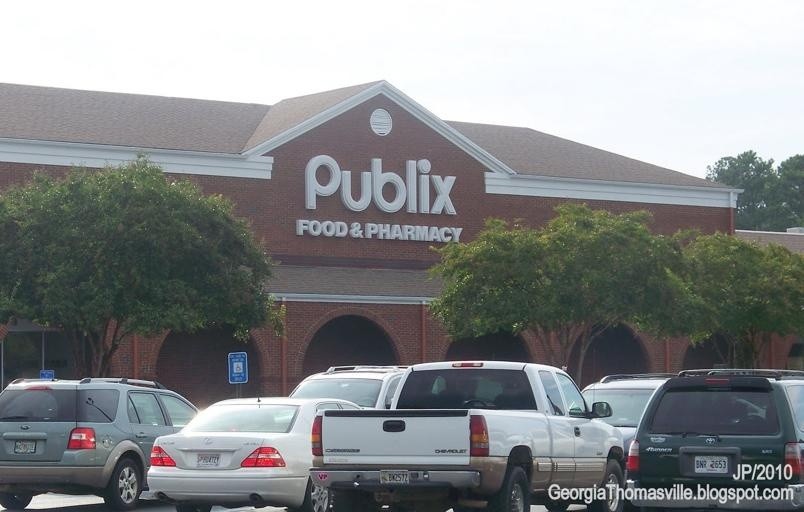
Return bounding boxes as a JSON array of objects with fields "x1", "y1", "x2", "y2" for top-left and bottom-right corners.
[{"x1": 428, "y1": 381, "x2": 528, "y2": 409}]
